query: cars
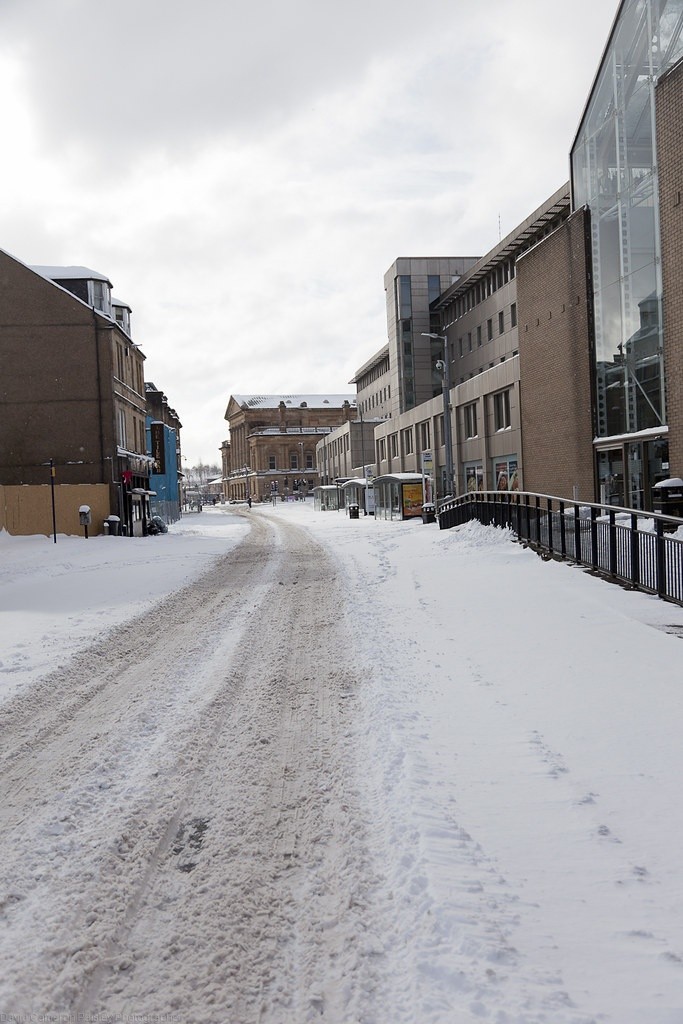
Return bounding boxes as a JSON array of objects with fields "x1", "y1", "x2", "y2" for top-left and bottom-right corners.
[{"x1": 230, "y1": 500, "x2": 247, "y2": 504}]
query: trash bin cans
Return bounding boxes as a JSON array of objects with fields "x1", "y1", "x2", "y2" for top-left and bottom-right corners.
[
  {"x1": 421, "y1": 506, "x2": 436, "y2": 524},
  {"x1": 103, "y1": 517, "x2": 119, "y2": 536},
  {"x1": 349, "y1": 505, "x2": 359, "y2": 519},
  {"x1": 651, "y1": 486, "x2": 683, "y2": 533}
]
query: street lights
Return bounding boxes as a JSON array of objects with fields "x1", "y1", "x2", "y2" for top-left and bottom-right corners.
[
  {"x1": 243, "y1": 463, "x2": 250, "y2": 499},
  {"x1": 342, "y1": 403, "x2": 367, "y2": 516},
  {"x1": 421, "y1": 331, "x2": 454, "y2": 498},
  {"x1": 298, "y1": 442, "x2": 305, "y2": 502}
]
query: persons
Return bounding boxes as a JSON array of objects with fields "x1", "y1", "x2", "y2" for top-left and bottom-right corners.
[{"x1": 213, "y1": 498, "x2": 216, "y2": 506}]
